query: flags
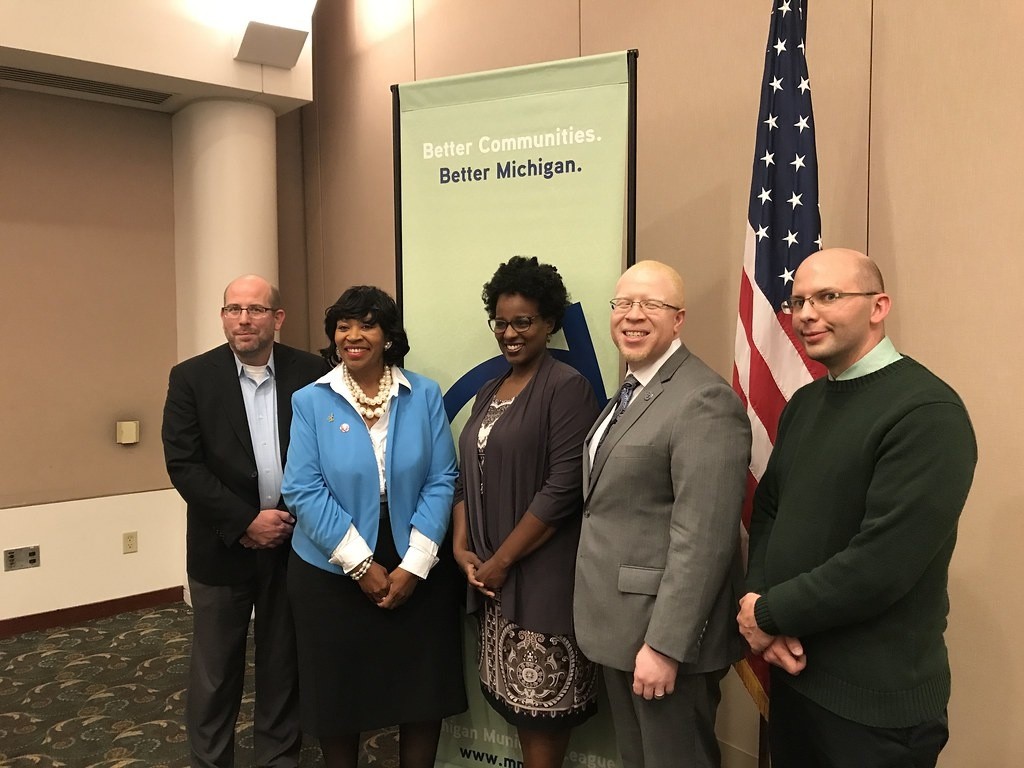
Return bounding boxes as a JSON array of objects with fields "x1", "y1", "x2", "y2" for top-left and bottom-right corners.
[
  {"x1": 738, "y1": 247, "x2": 978, "y2": 768},
  {"x1": 732, "y1": 1, "x2": 829, "y2": 725}
]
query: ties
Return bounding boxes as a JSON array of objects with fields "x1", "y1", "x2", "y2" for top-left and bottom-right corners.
[{"x1": 610, "y1": 374, "x2": 641, "y2": 425}]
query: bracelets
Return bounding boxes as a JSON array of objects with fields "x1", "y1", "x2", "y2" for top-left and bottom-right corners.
[{"x1": 350, "y1": 556, "x2": 373, "y2": 581}]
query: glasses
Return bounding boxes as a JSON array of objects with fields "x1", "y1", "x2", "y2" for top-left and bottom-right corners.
[
  {"x1": 610, "y1": 298, "x2": 679, "y2": 313},
  {"x1": 781, "y1": 289, "x2": 879, "y2": 314},
  {"x1": 488, "y1": 314, "x2": 540, "y2": 334},
  {"x1": 222, "y1": 304, "x2": 277, "y2": 319}
]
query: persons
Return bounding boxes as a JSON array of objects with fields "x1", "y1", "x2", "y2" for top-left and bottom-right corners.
[
  {"x1": 280, "y1": 285, "x2": 468, "y2": 767},
  {"x1": 452, "y1": 255, "x2": 598, "y2": 768},
  {"x1": 161, "y1": 274, "x2": 333, "y2": 768},
  {"x1": 571, "y1": 259, "x2": 749, "y2": 768}
]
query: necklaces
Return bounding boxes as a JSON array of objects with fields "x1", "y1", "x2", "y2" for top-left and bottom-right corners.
[{"x1": 340, "y1": 361, "x2": 395, "y2": 418}]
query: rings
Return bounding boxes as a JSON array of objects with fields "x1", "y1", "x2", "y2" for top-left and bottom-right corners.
[{"x1": 654, "y1": 692, "x2": 666, "y2": 700}]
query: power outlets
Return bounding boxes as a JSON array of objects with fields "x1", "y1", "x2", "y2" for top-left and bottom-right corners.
[{"x1": 123, "y1": 531, "x2": 138, "y2": 553}]
